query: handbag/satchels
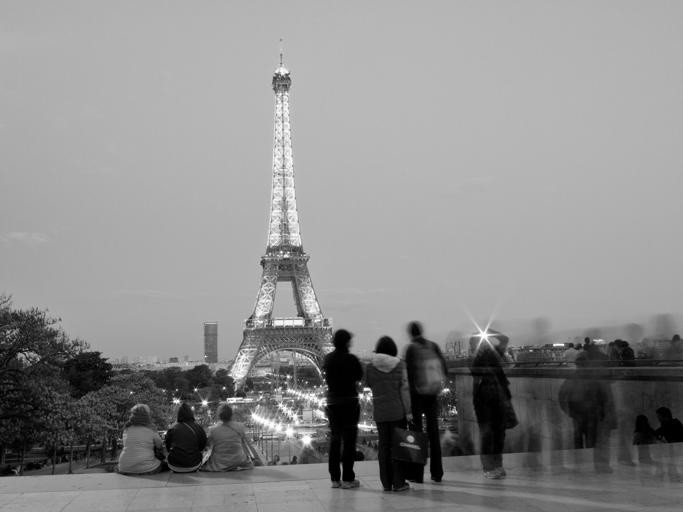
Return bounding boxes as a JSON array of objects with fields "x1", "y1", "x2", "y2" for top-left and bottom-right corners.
[
  {"x1": 390, "y1": 427, "x2": 428, "y2": 465},
  {"x1": 154, "y1": 444, "x2": 165, "y2": 459},
  {"x1": 502, "y1": 397, "x2": 518, "y2": 429}
]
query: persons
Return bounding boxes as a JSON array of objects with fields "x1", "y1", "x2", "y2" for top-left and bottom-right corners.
[
  {"x1": 116, "y1": 402, "x2": 169, "y2": 476},
  {"x1": 200, "y1": 403, "x2": 254, "y2": 473},
  {"x1": 522, "y1": 313, "x2": 683, "y2": 486},
  {"x1": 366, "y1": 335, "x2": 414, "y2": 493},
  {"x1": 467, "y1": 329, "x2": 520, "y2": 480},
  {"x1": 406, "y1": 321, "x2": 449, "y2": 483},
  {"x1": 268, "y1": 454, "x2": 308, "y2": 466},
  {"x1": 164, "y1": 403, "x2": 207, "y2": 473},
  {"x1": 325, "y1": 329, "x2": 364, "y2": 490}
]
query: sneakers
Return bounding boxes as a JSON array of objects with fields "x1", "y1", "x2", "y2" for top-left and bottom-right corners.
[
  {"x1": 495, "y1": 466, "x2": 505, "y2": 476},
  {"x1": 394, "y1": 483, "x2": 409, "y2": 491},
  {"x1": 332, "y1": 481, "x2": 342, "y2": 488},
  {"x1": 384, "y1": 488, "x2": 392, "y2": 491},
  {"x1": 342, "y1": 480, "x2": 359, "y2": 489},
  {"x1": 484, "y1": 470, "x2": 500, "y2": 479}
]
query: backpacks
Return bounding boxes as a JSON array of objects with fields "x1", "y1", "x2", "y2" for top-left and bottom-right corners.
[{"x1": 409, "y1": 340, "x2": 442, "y2": 395}]
